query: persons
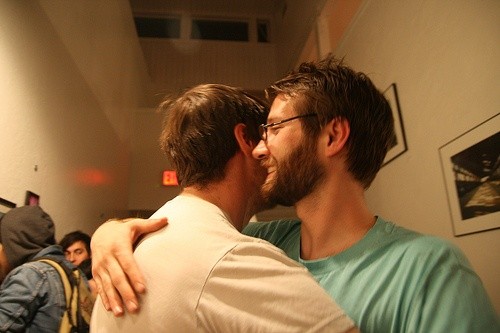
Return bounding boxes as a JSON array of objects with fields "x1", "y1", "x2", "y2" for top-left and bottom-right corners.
[
  {"x1": 90, "y1": 54, "x2": 500, "y2": 333},
  {"x1": 89, "y1": 84, "x2": 358, "y2": 333},
  {"x1": 0, "y1": 205, "x2": 99, "y2": 333}
]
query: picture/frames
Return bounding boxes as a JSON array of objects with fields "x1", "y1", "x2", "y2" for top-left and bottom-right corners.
[
  {"x1": 379, "y1": 83, "x2": 408, "y2": 168},
  {"x1": 437, "y1": 113, "x2": 500, "y2": 237},
  {"x1": 0, "y1": 191, "x2": 39, "y2": 220}
]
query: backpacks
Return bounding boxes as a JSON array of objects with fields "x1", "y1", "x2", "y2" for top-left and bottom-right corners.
[{"x1": 32, "y1": 257, "x2": 94, "y2": 333}]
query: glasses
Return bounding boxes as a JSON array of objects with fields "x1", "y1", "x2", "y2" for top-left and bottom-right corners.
[{"x1": 259, "y1": 113, "x2": 321, "y2": 140}]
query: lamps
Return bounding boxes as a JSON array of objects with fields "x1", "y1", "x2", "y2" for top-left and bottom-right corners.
[{"x1": 162, "y1": 170, "x2": 178, "y2": 188}]
